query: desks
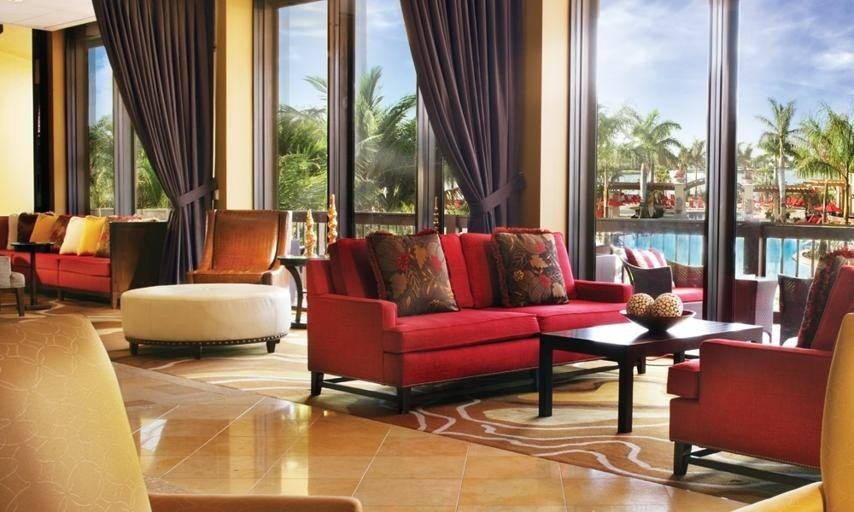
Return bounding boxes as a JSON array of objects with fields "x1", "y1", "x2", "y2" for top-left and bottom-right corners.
[
  {"x1": 735, "y1": 276, "x2": 779, "y2": 343},
  {"x1": 275, "y1": 256, "x2": 330, "y2": 330}
]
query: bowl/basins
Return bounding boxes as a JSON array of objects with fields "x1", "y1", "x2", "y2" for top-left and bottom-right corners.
[{"x1": 618, "y1": 309, "x2": 697, "y2": 340}]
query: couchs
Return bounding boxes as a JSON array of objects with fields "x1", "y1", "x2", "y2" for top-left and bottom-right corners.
[
  {"x1": 121, "y1": 282, "x2": 292, "y2": 359},
  {"x1": 733, "y1": 312, "x2": 852, "y2": 512},
  {"x1": 1, "y1": 215, "x2": 168, "y2": 309},
  {"x1": 622, "y1": 257, "x2": 704, "y2": 319},
  {"x1": 2, "y1": 314, "x2": 362, "y2": 512},
  {"x1": 304, "y1": 231, "x2": 647, "y2": 411},
  {"x1": 664, "y1": 264, "x2": 854, "y2": 485}
]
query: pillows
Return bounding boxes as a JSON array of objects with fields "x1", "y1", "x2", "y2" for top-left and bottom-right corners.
[
  {"x1": 50, "y1": 214, "x2": 73, "y2": 251},
  {"x1": 78, "y1": 215, "x2": 106, "y2": 254},
  {"x1": 778, "y1": 273, "x2": 814, "y2": 344},
  {"x1": 6, "y1": 213, "x2": 18, "y2": 250},
  {"x1": 625, "y1": 247, "x2": 676, "y2": 290},
  {"x1": 30, "y1": 214, "x2": 57, "y2": 243},
  {"x1": 96, "y1": 213, "x2": 142, "y2": 257},
  {"x1": 17, "y1": 212, "x2": 55, "y2": 243},
  {"x1": 797, "y1": 247, "x2": 854, "y2": 349},
  {"x1": 60, "y1": 216, "x2": 85, "y2": 255},
  {"x1": 366, "y1": 227, "x2": 459, "y2": 315},
  {"x1": 491, "y1": 226, "x2": 570, "y2": 306}
]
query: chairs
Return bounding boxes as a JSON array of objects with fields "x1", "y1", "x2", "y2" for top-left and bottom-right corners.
[
  {"x1": 188, "y1": 208, "x2": 294, "y2": 344},
  {"x1": 621, "y1": 259, "x2": 673, "y2": 299},
  {"x1": 0, "y1": 256, "x2": 26, "y2": 317}
]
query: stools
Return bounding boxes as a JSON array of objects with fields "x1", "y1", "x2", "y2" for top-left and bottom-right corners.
[{"x1": 10, "y1": 241, "x2": 54, "y2": 310}]
